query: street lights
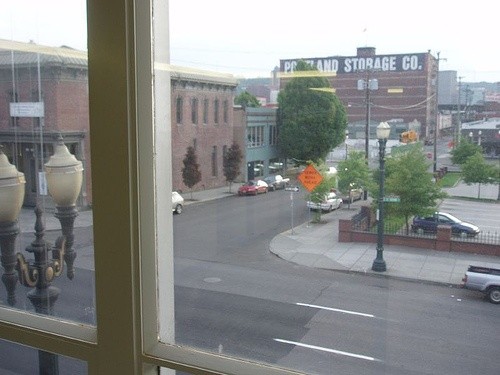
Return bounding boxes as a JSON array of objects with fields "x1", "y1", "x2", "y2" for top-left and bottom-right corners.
[
  {"x1": 370, "y1": 120, "x2": 391, "y2": 271},
  {"x1": 468, "y1": 132, "x2": 473, "y2": 144},
  {"x1": 0, "y1": 133, "x2": 85, "y2": 375}
]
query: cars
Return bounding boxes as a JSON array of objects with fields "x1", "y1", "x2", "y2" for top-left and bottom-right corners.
[
  {"x1": 325, "y1": 167, "x2": 337, "y2": 177},
  {"x1": 338, "y1": 182, "x2": 363, "y2": 202},
  {"x1": 306, "y1": 191, "x2": 344, "y2": 213},
  {"x1": 171, "y1": 191, "x2": 185, "y2": 214},
  {"x1": 411, "y1": 212, "x2": 481, "y2": 237},
  {"x1": 238, "y1": 179, "x2": 269, "y2": 195}
]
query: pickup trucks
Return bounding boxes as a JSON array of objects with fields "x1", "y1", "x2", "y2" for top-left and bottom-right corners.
[
  {"x1": 462, "y1": 265, "x2": 500, "y2": 304},
  {"x1": 263, "y1": 175, "x2": 290, "y2": 191}
]
